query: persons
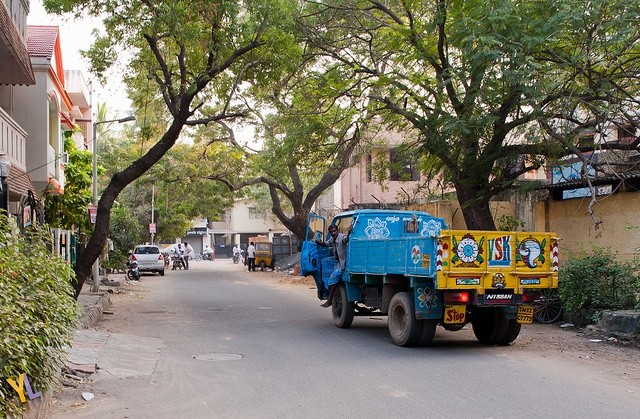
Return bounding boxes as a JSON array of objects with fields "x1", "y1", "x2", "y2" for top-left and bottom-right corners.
[
  {"x1": 311, "y1": 224, "x2": 354, "y2": 308},
  {"x1": 176, "y1": 242, "x2": 191, "y2": 270},
  {"x1": 246, "y1": 242, "x2": 256, "y2": 273},
  {"x1": 201, "y1": 245, "x2": 215, "y2": 261},
  {"x1": 231, "y1": 242, "x2": 244, "y2": 263}
]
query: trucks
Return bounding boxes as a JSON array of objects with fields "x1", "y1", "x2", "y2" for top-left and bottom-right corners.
[
  {"x1": 254, "y1": 241, "x2": 275, "y2": 271},
  {"x1": 299, "y1": 208, "x2": 563, "y2": 346}
]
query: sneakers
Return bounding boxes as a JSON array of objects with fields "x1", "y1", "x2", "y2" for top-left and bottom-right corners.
[
  {"x1": 171, "y1": 268, "x2": 174, "y2": 270},
  {"x1": 184, "y1": 266, "x2": 189, "y2": 270}
]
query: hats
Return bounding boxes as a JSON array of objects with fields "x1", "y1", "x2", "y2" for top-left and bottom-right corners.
[{"x1": 234, "y1": 243, "x2": 238, "y2": 246}]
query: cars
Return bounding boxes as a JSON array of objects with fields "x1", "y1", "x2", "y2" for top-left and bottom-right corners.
[{"x1": 132, "y1": 245, "x2": 165, "y2": 276}]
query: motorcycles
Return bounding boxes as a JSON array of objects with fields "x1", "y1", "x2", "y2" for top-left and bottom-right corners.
[
  {"x1": 232, "y1": 250, "x2": 244, "y2": 264},
  {"x1": 171, "y1": 250, "x2": 183, "y2": 270},
  {"x1": 126, "y1": 260, "x2": 141, "y2": 281},
  {"x1": 195, "y1": 248, "x2": 215, "y2": 261},
  {"x1": 161, "y1": 252, "x2": 170, "y2": 267}
]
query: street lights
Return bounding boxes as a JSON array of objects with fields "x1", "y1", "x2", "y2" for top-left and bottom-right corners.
[{"x1": 91, "y1": 117, "x2": 136, "y2": 293}]
